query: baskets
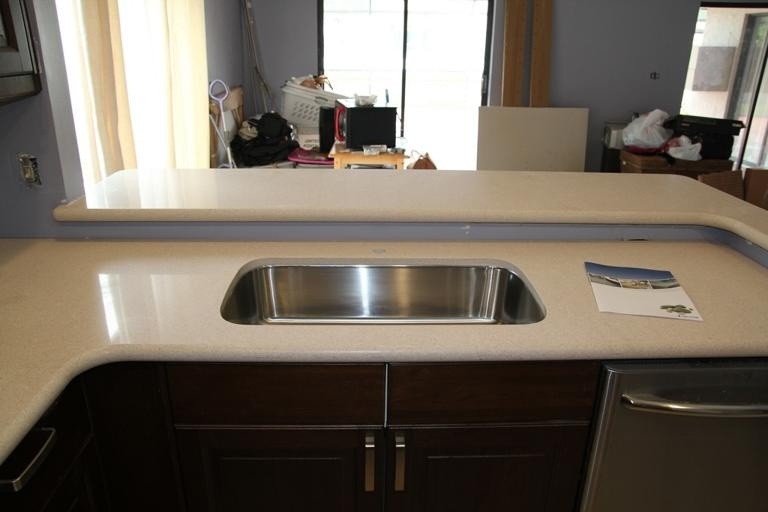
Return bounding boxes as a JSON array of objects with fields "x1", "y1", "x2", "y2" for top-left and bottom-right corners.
[{"x1": 277, "y1": 78, "x2": 352, "y2": 128}]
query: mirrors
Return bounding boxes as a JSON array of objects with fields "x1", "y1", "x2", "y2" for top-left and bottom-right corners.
[{"x1": 203, "y1": 2, "x2": 768, "y2": 208}]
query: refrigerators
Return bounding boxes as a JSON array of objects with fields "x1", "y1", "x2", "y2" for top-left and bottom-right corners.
[{"x1": 575, "y1": 365, "x2": 768, "y2": 509}]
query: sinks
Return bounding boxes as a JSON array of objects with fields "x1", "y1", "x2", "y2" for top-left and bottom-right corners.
[{"x1": 219, "y1": 257, "x2": 548, "y2": 326}]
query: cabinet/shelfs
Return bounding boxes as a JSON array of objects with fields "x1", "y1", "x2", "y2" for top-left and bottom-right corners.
[
  {"x1": 615, "y1": 147, "x2": 733, "y2": 180},
  {"x1": 90, "y1": 363, "x2": 601, "y2": 510},
  {"x1": 0, "y1": 379, "x2": 105, "y2": 510}
]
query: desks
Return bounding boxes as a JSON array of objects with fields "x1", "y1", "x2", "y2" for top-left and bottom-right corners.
[{"x1": 328, "y1": 141, "x2": 412, "y2": 170}]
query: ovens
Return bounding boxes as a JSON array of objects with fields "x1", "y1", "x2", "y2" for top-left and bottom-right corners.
[{"x1": 334, "y1": 94, "x2": 397, "y2": 152}]
